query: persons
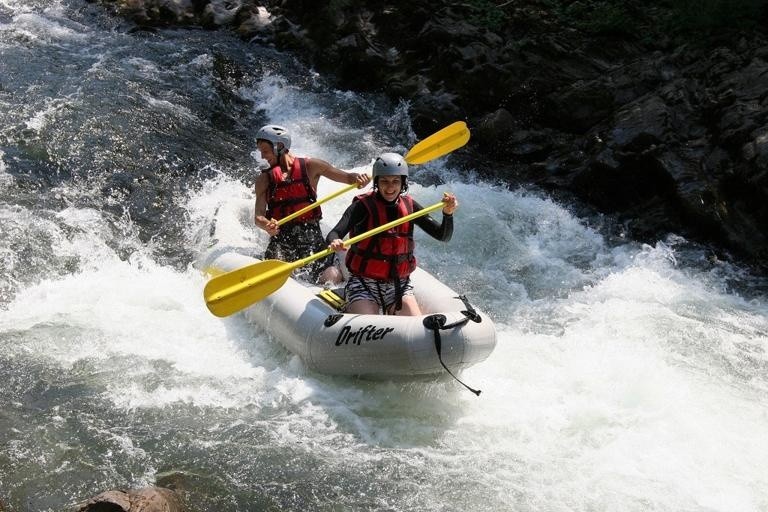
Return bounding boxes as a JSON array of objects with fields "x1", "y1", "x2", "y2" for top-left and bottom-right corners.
[
  {"x1": 324, "y1": 151, "x2": 459, "y2": 317},
  {"x1": 252, "y1": 124, "x2": 372, "y2": 286}
]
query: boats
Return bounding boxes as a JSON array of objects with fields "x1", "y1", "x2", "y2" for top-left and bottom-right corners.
[{"x1": 194, "y1": 195, "x2": 499, "y2": 397}]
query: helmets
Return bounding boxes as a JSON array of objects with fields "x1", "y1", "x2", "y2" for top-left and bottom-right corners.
[
  {"x1": 372, "y1": 152, "x2": 409, "y2": 176},
  {"x1": 256, "y1": 125, "x2": 291, "y2": 149}
]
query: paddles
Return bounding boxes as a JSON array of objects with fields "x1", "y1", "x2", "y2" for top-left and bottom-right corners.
[
  {"x1": 203, "y1": 193, "x2": 450, "y2": 317},
  {"x1": 271, "y1": 121, "x2": 470, "y2": 228}
]
